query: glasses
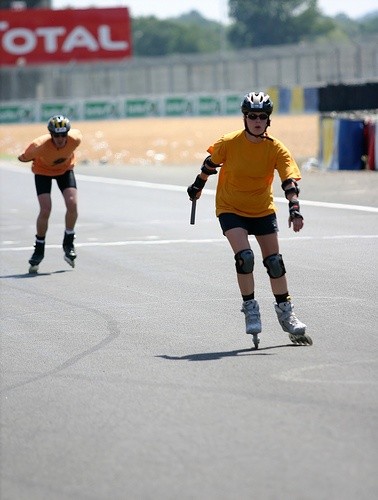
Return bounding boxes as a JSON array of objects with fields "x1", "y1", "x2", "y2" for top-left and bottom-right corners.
[
  {"x1": 51, "y1": 132, "x2": 67, "y2": 137},
  {"x1": 246, "y1": 113, "x2": 269, "y2": 120}
]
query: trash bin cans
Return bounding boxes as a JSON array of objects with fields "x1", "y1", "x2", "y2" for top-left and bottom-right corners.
[{"x1": 338, "y1": 117, "x2": 376, "y2": 170}]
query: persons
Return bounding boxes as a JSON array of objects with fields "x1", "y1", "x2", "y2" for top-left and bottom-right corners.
[
  {"x1": 18, "y1": 114, "x2": 81, "y2": 274},
  {"x1": 187, "y1": 92, "x2": 313, "y2": 350}
]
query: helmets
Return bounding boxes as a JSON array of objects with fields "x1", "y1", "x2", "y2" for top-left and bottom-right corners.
[
  {"x1": 47, "y1": 115, "x2": 71, "y2": 133},
  {"x1": 240, "y1": 91, "x2": 273, "y2": 115}
]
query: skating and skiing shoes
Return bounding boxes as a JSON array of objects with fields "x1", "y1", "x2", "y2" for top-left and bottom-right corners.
[
  {"x1": 62, "y1": 232, "x2": 77, "y2": 268},
  {"x1": 29, "y1": 241, "x2": 45, "y2": 273},
  {"x1": 241, "y1": 299, "x2": 262, "y2": 349},
  {"x1": 274, "y1": 296, "x2": 313, "y2": 345}
]
